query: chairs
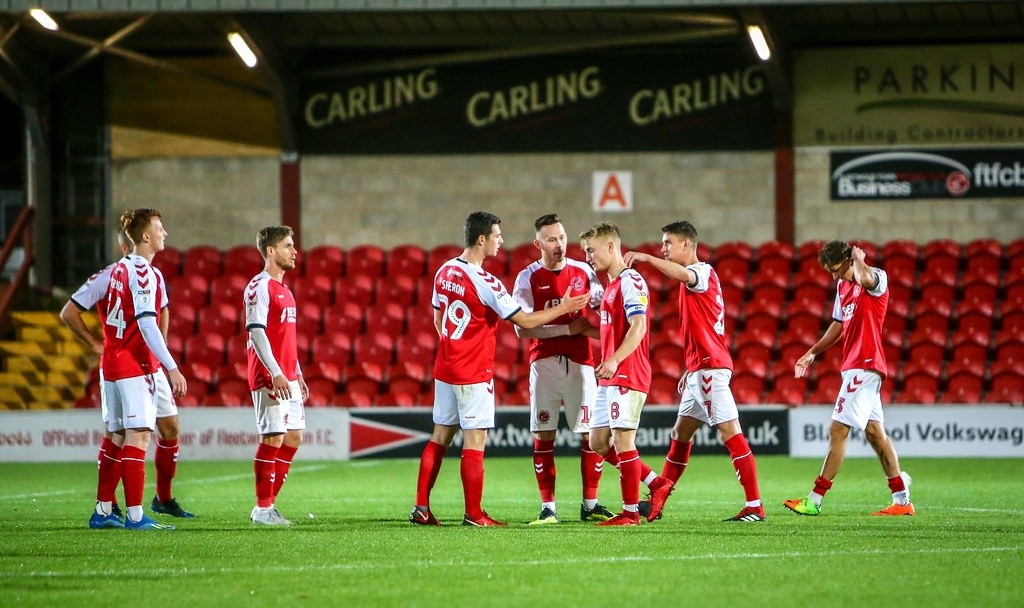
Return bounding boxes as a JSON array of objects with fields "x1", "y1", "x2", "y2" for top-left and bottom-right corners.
[{"x1": 0, "y1": 237, "x2": 1024, "y2": 412}]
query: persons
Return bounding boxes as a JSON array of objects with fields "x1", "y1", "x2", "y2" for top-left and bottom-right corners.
[
  {"x1": 59, "y1": 208, "x2": 197, "y2": 530},
  {"x1": 244, "y1": 226, "x2": 308, "y2": 527},
  {"x1": 511, "y1": 214, "x2": 676, "y2": 527},
  {"x1": 784, "y1": 240, "x2": 916, "y2": 516},
  {"x1": 410, "y1": 211, "x2": 591, "y2": 528},
  {"x1": 623, "y1": 220, "x2": 768, "y2": 523}
]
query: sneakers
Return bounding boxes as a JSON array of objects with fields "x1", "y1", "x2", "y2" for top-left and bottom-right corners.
[
  {"x1": 594, "y1": 510, "x2": 641, "y2": 527},
  {"x1": 723, "y1": 503, "x2": 767, "y2": 523},
  {"x1": 150, "y1": 494, "x2": 193, "y2": 519},
  {"x1": 528, "y1": 504, "x2": 560, "y2": 525},
  {"x1": 409, "y1": 506, "x2": 441, "y2": 526},
  {"x1": 580, "y1": 502, "x2": 617, "y2": 521},
  {"x1": 463, "y1": 509, "x2": 507, "y2": 527},
  {"x1": 638, "y1": 500, "x2": 663, "y2": 519},
  {"x1": 644, "y1": 475, "x2": 674, "y2": 522},
  {"x1": 124, "y1": 513, "x2": 176, "y2": 531},
  {"x1": 111, "y1": 503, "x2": 125, "y2": 521},
  {"x1": 870, "y1": 503, "x2": 915, "y2": 517},
  {"x1": 783, "y1": 496, "x2": 821, "y2": 516},
  {"x1": 89, "y1": 511, "x2": 124, "y2": 529},
  {"x1": 251, "y1": 505, "x2": 292, "y2": 525}
]
